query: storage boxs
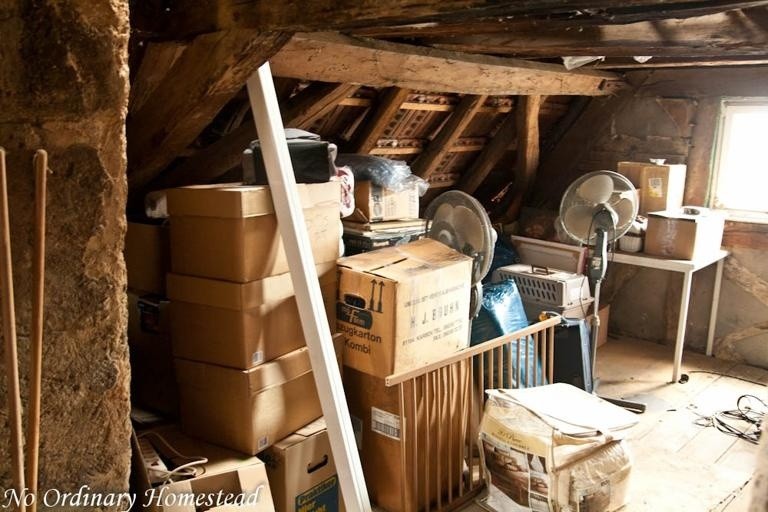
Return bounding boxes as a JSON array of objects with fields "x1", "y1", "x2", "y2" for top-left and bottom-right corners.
[
  {"x1": 260, "y1": 415, "x2": 341, "y2": 512},
  {"x1": 336, "y1": 234, "x2": 471, "y2": 379},
  {"x1": 349, "y1": 178, "x2": 418, "y2": 222},
  {"x1": 166, "y1": 177, "x2": 340, "y2": 283},
  {"x1": 132, "y1": 427, "x2": 274, "y2": 512},
  {"x1": 647, "y1": 207, "x2": 725, "y2": 258},
  {"x1": 619, "y1": 162, "x2": 686, "y2": 213},
  {"x1": 166, "y1": 260, "x2": 340, "y2": 369},
  {"x1": 175, "y1": 332, "x2": 345, "y2": 456},
  {"x1": 342, "y1": 365, "x2": 462, "y2": 512}
]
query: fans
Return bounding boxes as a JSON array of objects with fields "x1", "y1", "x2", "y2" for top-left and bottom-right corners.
[
  {"x1": 422, "y1": 191, "x2": 495, "y2": 326},
  {"x1": 560, "y1": 169, "x2": 645, "y2": 407}
]
